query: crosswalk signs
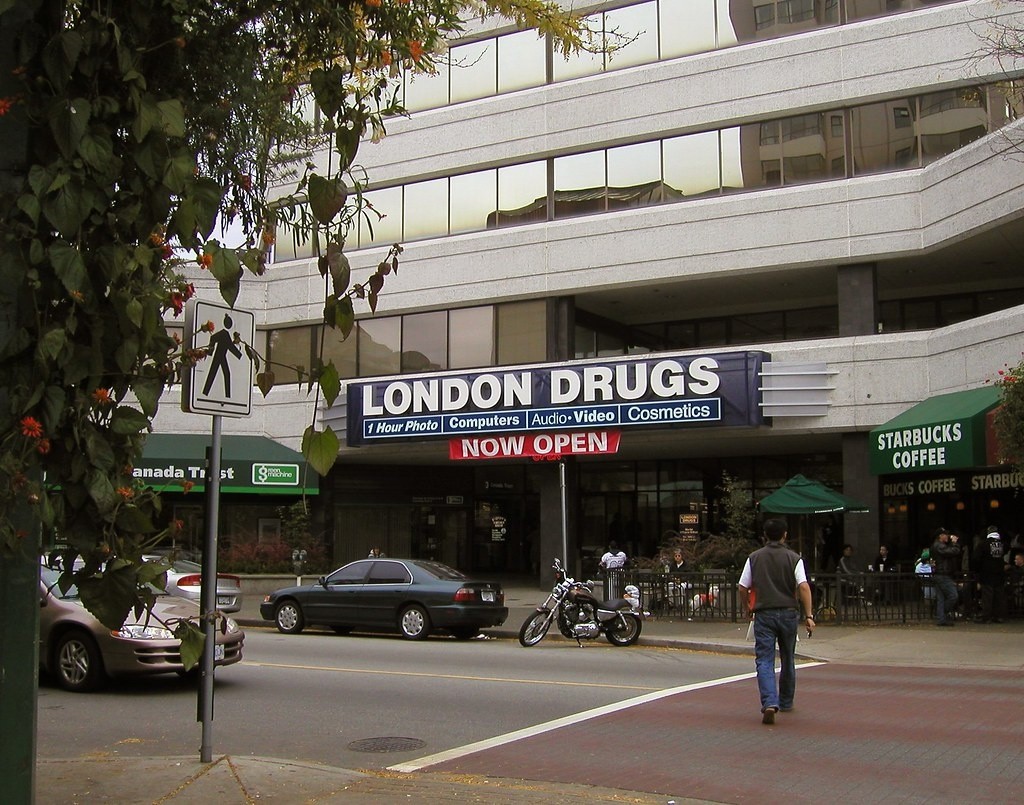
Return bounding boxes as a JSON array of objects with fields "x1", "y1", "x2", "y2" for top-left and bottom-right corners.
[{"x1": 181, "y1": 298, "x2": 256, "y2": 419}]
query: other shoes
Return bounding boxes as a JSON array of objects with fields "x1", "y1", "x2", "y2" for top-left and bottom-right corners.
[
  {"x1": 779, "y1": 706, "x2": 792, "y2": 712},
  {"x1": 937, "y1": 622, "x2": 954, "y2": 627},
  {"x1": 762, "y1": 709, "x2": 775, "y2": 724}
]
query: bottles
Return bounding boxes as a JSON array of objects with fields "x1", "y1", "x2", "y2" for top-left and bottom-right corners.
[{"x1": 665, "y1": 565, "x2": 669, "y2": 573}]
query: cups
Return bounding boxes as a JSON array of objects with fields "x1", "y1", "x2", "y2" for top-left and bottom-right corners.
[
  {"x1": 950, "y1": 535, "x2": 959, "y2": 540},
  {"x1": 880, "y1": 564, "x2": 884, "y2": 572},
  {"x1": 868, "y1": 565, "x2": 872, "y2": 570}
]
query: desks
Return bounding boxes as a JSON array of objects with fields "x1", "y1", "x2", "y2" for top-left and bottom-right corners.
[
  {"x1": 868, "y1": 572, "x2": 915, "y2": 624},
  {"x1": 952, "y1": 580, "x2": 978, "y2": 624},
  {"x1": 809, "y1": 573, "x2": 867, "y2": 624},
  {"x1": 671, "y1": 571, "x2": 730, "y2": 622}
]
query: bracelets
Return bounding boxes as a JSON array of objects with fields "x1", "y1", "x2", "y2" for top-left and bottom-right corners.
[{"x1": 806, "y1": 615, "x2": 813, "y2": 619}]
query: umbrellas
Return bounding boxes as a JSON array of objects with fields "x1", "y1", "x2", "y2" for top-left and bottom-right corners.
[{"x1": 756, "y1": 473, "x2": 871, "y2": 555}]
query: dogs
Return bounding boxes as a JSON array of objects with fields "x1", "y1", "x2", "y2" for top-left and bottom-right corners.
[{"x1": 687, "y1": 586, "x2": 720, "y2": 617}]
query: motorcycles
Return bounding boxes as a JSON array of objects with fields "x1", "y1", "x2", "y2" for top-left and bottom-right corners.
[{"x1": 519, "y1": 558, "x2": 645, "y2": 646}]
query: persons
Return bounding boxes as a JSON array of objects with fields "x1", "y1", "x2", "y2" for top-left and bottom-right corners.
[
  {"x1": 835, "y1": 545, "x2": 873, "y2": 607},
  {"x1": 874, "y1": 544, "x2": 897, "y2": 573},
  {"x1": 608, "y1": 512, "x2": 624, "y2": 548},
  {"x1": 424, "y1": 537, "x2": 437, "y2": 562},
  {"x1": 738, "y1": 518, "x2": 815, "y2": 725},
  {"x1": 664, "y1": 549, "x2": 689, "y2": 605},
  {"x1": 368, "y1": 545, "x2": 387, "y2": 560},
  {"x1": 814, "y1": 514, "x2": 832, "y2": 573},
  {"x1": 599, "y1": 541, "x2": 627, "y2": 600},
  {"x1": 914, "y1": 525, "x2": 1024, "y2": 626}
]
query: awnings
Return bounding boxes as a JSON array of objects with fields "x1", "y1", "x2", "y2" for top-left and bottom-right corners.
[
  {"x1": 43, "y1": 433, "x2": 319, "y2": 495},
  {"x1": 869, "y1": 384, "x2": 1024, "y2": 476}
]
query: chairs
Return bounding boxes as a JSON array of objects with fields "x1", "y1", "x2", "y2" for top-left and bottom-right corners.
[{"x1": 638, "y1": 563, "x2": 1024, "y2": 624}]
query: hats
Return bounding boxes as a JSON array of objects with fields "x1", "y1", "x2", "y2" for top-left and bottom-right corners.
[{"x1": 934, "y1": 528, "x2": 949, "y2": 536}]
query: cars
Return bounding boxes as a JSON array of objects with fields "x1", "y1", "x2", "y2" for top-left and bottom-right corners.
[
  {"x1": 260, "y1": 560, "x2": 508, "y2": 640},
  {"x1": 36, "y1": 549, "x2": 246, "y2": 688}
]
